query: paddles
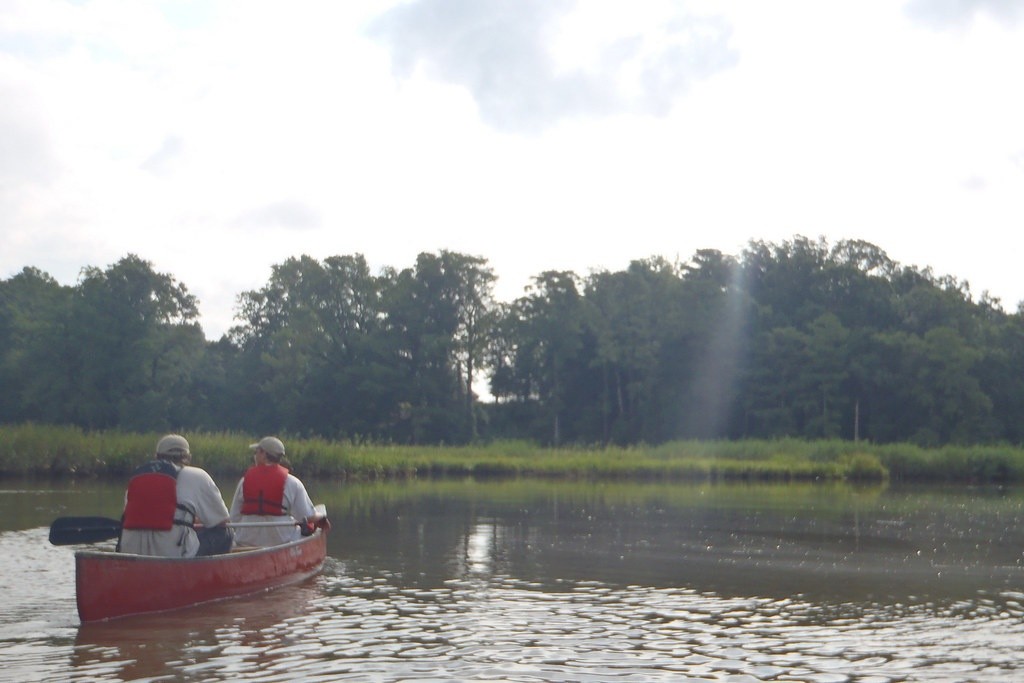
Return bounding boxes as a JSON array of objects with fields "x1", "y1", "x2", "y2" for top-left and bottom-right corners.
[{"x1": 48, "y1": 514, "x2": 308, "y2": 546}]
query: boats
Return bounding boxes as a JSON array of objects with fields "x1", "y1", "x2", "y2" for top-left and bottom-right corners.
[{"x1": 74, "y1": 501, "x2": 330, "y2": 626}]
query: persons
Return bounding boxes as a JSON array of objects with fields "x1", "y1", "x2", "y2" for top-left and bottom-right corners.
[
  {"x1": 121, "y1": 435, "x2": 233, "y2": 558},
  {"x1": 227, "y1": 438, "x2": 313, "y2": 547}
]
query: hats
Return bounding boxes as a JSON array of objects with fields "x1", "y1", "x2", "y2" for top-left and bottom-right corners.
[
  {"x1": 156, "y1": 434, "x2": 190, "y2": 457},
  {"x1": 248, "y1": 436, "x2": 285, "y2": 458}
]
